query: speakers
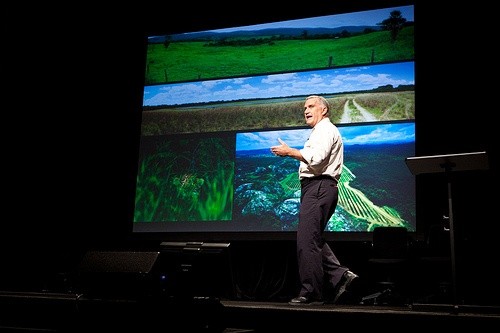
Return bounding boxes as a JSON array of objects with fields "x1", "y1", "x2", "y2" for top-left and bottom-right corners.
[{"x1": 72, "y1": 251, "x2": 174, "y2": 302}]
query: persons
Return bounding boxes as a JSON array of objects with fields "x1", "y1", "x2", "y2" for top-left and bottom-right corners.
[{"x1": 271, "y1": 95, "x2": 360, "y2": 306}]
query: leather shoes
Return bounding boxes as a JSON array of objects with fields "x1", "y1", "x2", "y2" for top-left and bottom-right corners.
[
  {"x1": 288, "y1": 294, "x2": 326, "y2": 306},
  {"x1": 333, "y1": 271, "x2": 359, "y2": 305}
]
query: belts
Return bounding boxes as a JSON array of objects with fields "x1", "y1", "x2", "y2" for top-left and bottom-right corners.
[{"x1": 301, "y1": 175, "x2": 337, "y2": 186}]
